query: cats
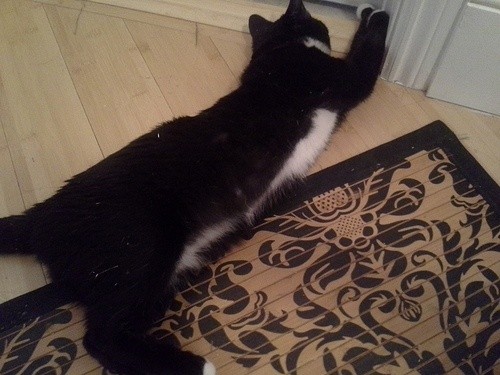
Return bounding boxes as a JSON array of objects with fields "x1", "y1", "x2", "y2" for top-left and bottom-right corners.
[{"x1": 0, "y1": 0, "x2": 391, "y2": 375}]
